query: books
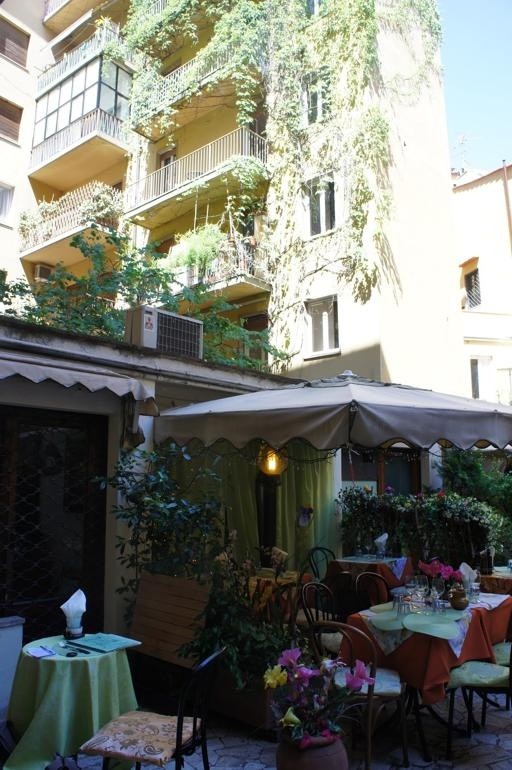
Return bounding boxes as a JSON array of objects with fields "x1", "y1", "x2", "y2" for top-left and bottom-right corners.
[{"x1": 66, "y1": 632, "x2": 142, "y2": 652}]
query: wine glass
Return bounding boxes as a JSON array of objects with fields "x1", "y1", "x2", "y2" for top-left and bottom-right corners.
[
  {"x1": 405, "y1": 574, "x2": 444, "y2": 611},
  {"x1": 469, "y1": 571, "x2": 481, "y2": 603},
  {"x1": 363, "y1": 539, "x2": 373, "y2": 558}
]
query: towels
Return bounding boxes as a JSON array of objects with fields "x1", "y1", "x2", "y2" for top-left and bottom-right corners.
[
  {"x1": 59, "y1": 590, "x2": 86, "y2": 628},
  {"x1": 375, "y1": 533, "x2": 388, "y2": 551}
]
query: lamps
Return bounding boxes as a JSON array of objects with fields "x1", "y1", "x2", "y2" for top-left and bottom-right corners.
[{"x1": 256, "y1": 444, "x2": 289, "y2": 488}]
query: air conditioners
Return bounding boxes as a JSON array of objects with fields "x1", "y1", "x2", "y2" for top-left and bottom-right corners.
[{"x1": 123, "y1": 304, "x2": 204, "y2": 361}]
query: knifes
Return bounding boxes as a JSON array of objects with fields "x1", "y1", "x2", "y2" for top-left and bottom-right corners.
[{"x1": 65, "y1": 645, "x2": 90, "y2": 655}]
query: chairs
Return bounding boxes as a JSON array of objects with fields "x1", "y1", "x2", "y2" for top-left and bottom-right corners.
[
  {"x1": 263, "y1": 548, "x2": 512, "y2": 770},
  {"x1": 77, "y1": 644, "x2": 228, "y2": 770}
]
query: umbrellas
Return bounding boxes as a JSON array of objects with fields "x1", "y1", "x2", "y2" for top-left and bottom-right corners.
[{"x1": 154, "y1": 371, "x2": 512, "y2": 486}]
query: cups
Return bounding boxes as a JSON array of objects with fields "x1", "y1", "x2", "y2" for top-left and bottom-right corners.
[{"x1": 392, "y1": 593, "x2": 409, "y2": 616}]
